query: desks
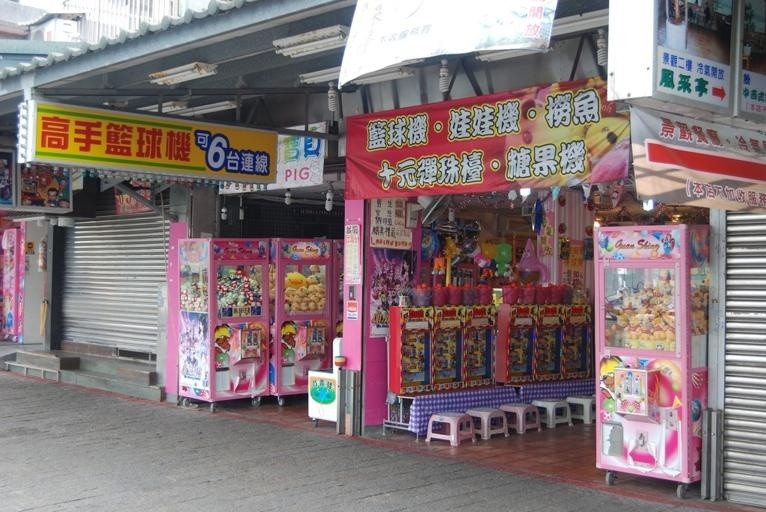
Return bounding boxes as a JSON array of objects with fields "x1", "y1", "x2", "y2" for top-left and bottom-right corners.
[
  {"x1": 382, "y1": 385, "x2": 517, "y2": 443},
  {"x1": 505, "y1": 379, "x2": 595, "y2": 402}
]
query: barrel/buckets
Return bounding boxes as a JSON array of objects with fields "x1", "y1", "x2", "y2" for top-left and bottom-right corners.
[
  {"x1": 502, "y1": 286, "x2": 517, "y2": 306},
  {"x1": 476, "y1": 287, "x2": 491, "y2": 306},
  {"x1": 535, "y1": 287, "x2": 547, "y2": 306},
  {"x1": 431, "y1": 288, "x2": 447, "y2": 307},
  {"x1": 517, "y1": 288, "x2": 534, "y2": 305},
  {"x1": 461, "y1": 287, "x2": 477, "y2": 306},
  {"x1": 546, "y1": 286, "x2": 564, "y2": 305},
  {"x1": 412, "y1": 287, "x2": 430, "y2": 307},
  {"x1": 446, "y1": 287, "x2": 461, "y2": 306}
]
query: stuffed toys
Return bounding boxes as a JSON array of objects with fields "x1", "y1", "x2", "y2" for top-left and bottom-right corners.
[
  {"x1": 606, "y1": 282, "x2": 709, "y2": 351},
  {"x1": 269, "y1": 265, "x2": 326, "y2": 311}
]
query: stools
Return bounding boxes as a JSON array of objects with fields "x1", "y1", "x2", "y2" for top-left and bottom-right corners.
[{"x1": 426, "y1": 392, "x2": 594, "y2": 448}]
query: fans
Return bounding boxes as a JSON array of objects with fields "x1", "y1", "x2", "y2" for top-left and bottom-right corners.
[{"x1": 404, "y1": 189, "x2": 452, "y2": 229}]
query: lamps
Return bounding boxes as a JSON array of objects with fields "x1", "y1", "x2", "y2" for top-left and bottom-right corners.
[
  {"x1": 283, "y1": 188, "x2": 291, "y2": 205},
  {"x1": 297, "y1": 53, "x2": 425, "y2": 81},
  {"x1": 550, "y1": 8, "x2": 609, "y2": 37},
  {"x1": 438, "y1": 58, "x2": 449, "y2": 91},
  {"x1": 271, "y1": 24, "x2": 349, "y2": 59},
  {"x1": 349, "y1": 67, "x2": 415, "y2": 83},
  {"x1": 147, "y1": 61, "x2": 216, "y2": 86},
  {"x1": 595, "y1": 25, "x2": 607, "y2": 66},
  {"x1": 165, "y1": 100, "x2": 242, "y2": 116},
  {"x1": 326, "y1": 81, "x2": 337, "y2": 112},
  {"x1": 323, "y1": 180, "x2": 335, "y2": 212},
  {"x1": 474, "y1": 50, "x2": 542, "y2": 64},
  {"x1": 220, "y1": 196, "x2": 228, "y2": 222},
  {"x1": 135, "y1": 100, "x2": 187, "y2": 114}
]
